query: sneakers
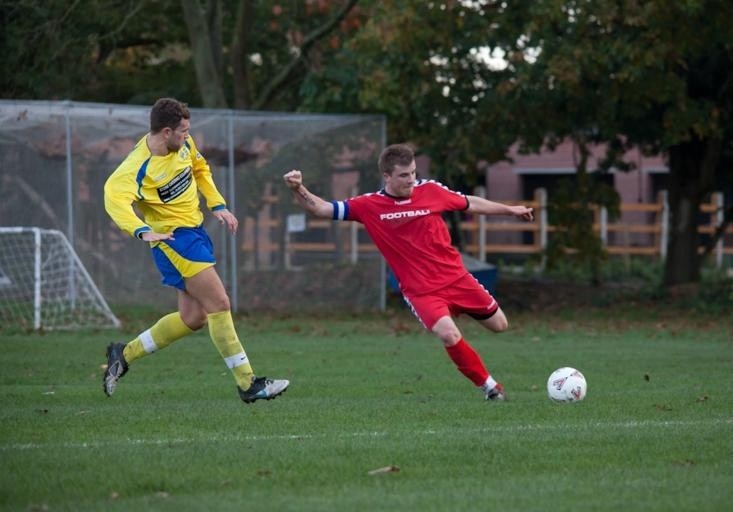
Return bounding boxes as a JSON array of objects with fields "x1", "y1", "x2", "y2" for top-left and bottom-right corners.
[
  {"x1": 103, "y1": 342, "x2": 129, "y2": 398},
  {"x1": 237, "y1": 376, "x2": 290, "y2": 404},
  {"x1": 484, "y1": 383, "x2": 506, "y2": 402}
]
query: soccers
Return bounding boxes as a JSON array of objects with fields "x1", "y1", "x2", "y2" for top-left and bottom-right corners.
[{"x1": 547, "y1": 367, "x2": 587, "y2": 404}]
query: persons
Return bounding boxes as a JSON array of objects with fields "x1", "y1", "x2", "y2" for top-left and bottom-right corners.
[
  {"x1": 100, "y1": 96, "x2": 292, "y2": 403},
  {"x1": 281, "y1": 142, "x2": 536, "y2": 403}
]
